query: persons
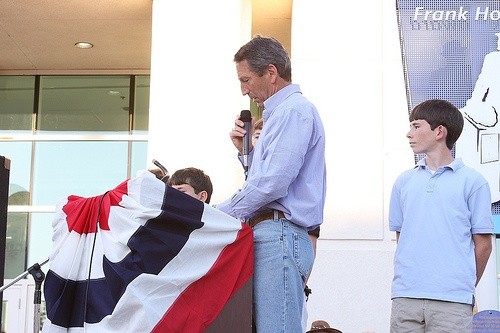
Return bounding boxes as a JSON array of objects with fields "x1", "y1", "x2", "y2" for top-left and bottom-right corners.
[
  {"x1": 227, "y1": 35, "x2": 327, "y2": 333},
  {"x1": 388, "y1": 98, "x2": 494, "y2": 332},
  {"x1": 170, "y1": 167, "x2": 215, "y2": 207},
  {"x1": 252, "y1": 118, "x2": 322, "y2": 333}
]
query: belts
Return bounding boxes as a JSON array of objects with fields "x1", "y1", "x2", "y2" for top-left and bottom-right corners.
[{"x1": 248, "y1": 211, "x2": 285, "y2": 227}]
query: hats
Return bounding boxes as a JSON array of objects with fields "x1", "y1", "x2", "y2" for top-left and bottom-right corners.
[{"x1": 306, "y1": 320, "x2": 343, "y2": 333}]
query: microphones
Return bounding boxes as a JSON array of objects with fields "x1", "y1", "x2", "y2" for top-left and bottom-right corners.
[{"x1": 240, "y1": 109, "x2": 252, "y2": 177}]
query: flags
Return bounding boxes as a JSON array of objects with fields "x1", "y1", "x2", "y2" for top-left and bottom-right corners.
[{"x1": 39, "y1": 171, "x2": 257, "y2": 332}]
